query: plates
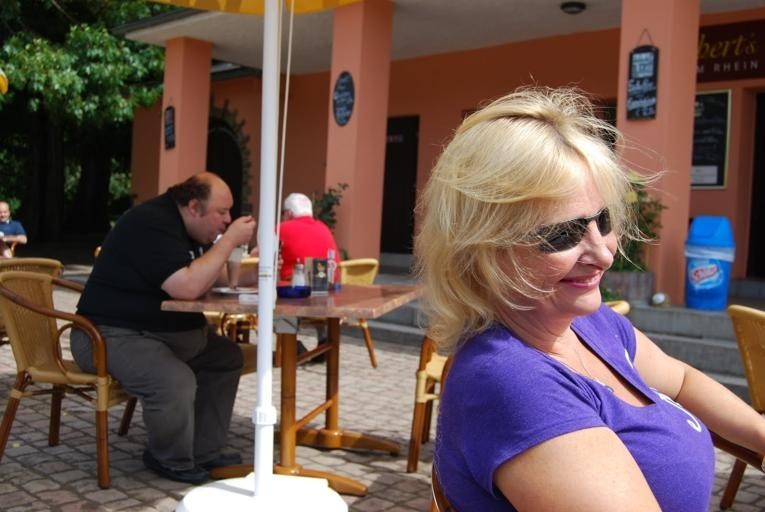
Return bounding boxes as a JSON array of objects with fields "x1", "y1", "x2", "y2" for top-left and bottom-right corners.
[{"x1": 211, "y1": 284, "x2": 261, "y2": 298}]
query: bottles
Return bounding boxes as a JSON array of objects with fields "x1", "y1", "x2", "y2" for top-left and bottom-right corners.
[{"x1": 291, "y1": 263, "x2": 305, "y2": 288}]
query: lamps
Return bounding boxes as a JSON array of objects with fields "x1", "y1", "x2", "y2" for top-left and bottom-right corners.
[{"x1": 561, "y1": 2, "x2": 585, "y2": 15}]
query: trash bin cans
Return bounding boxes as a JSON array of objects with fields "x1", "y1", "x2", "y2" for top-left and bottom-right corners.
[{"x1": 684, "y1": 215, "x2": 737, "y2": 310}]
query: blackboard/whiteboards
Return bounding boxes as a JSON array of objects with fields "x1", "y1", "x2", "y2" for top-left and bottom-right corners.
[
  {"x1": 332, "y1": 70, "x2": 355, "y2": 127},
  {"x1": 164, "y1": 106, "x2": 175, "y2": 150},
  {"x1": 627, "y1": 45, "x2": 659, "y2": 120}
]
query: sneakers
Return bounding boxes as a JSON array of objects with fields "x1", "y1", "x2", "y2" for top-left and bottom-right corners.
[
  {"x1": 204, "y1": 452, "x2": 242, "y2": 471},
  {"x1": 142, "y1": 458, "x2": 209, "y2": 483}
]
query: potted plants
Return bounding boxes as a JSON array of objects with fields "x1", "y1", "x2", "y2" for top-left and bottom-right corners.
[{"x1": 597, "y1": 175, "x2": 668, "y2": 304}]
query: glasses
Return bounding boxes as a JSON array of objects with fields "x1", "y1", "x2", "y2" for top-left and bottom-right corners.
[{"x1": 521, "y1": 203, "x2": 613, "y2": 254}]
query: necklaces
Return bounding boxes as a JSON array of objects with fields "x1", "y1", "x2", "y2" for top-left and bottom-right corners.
[{"x1": 553, "y1": 346, "x2": 618, "y2": 394}]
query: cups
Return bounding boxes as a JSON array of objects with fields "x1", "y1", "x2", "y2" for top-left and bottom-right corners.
[{"x1": 227, "y1": 247, "x2": 243, "y2": 290}]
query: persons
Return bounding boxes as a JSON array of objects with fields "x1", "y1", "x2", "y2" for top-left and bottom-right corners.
[
  {"x1": 249, "y1": 191, "x2": 343, "y2": 366},
  {"x1": 412, "y1": 83, "x2": 765, "y2": 512},
  {"x1": 0, "y1": 201, "x2": 27, "y2": 261},
  {"x1": 69, "y1": 170, "x2": 257, "y2": 484}
]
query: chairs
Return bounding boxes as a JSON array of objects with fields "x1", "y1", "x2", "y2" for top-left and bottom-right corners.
[
  {"x1": 407, "y1": 300, "x2": 632, "y2": 473},
  {"x1": 0, "y1": 271, "x2": 138, "y2": 489},
  {"x1": 275, "y1": 257, "x2": 379, "y2": 368},
  {"x1": 204, "y1": 255, "x2": 260, "y2": 342},
  {"x1": 0, "y1": 257, "x2": 64, "y2": 348},
  {"x1": 720, "y1": 304, "x2": 765, "y2": 509},
  {"x1": 428, "y1": 350, "x2": 765, "y2": 512}
]
